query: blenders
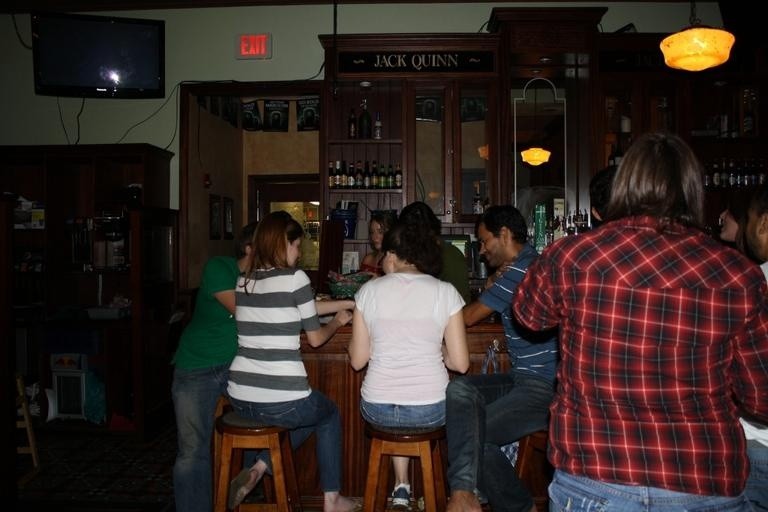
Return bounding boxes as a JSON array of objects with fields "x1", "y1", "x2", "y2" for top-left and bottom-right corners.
[{"x1": 468, "y1": 242, "x2": 499, "y2": 324}]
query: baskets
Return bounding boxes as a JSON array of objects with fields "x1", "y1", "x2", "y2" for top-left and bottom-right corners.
[{"x1": 327, "y1": 272, "x2": 373, "y2": 297}]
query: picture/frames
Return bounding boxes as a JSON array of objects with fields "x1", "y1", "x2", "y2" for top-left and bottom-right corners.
[
  {"x1": 209, "y1": 194, "x2": 222, "y2": 240},
  {"x1": 224, "y1": 197, "x2": 234, "y2": 240}
]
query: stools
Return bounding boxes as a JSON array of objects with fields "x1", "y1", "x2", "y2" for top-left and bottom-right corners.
[
  {"x1": 214, "y1": 411, "x2": 303, "y2": 512},
  {"x1": 363, "y1": 423, "x2": 448, "y2": 512},
  {"x1": 515, "y1": 431, "x2": 551, "y2": 506}
]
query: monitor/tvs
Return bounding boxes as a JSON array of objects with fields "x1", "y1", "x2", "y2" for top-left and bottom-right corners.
[{"x1": 30, "y1": 12, "x2": 165, "y2": 99}]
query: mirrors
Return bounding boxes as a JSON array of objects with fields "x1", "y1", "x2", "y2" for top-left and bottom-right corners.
[
  {"x1": 460, "y1": 86, "x2": 493, "y2": 215},
  {"x1": 415, "y1": 86, "x2": 445, "y2": 215},
  {"x1": 514, "y1": 88, "x2": 567, "y2": 231}
]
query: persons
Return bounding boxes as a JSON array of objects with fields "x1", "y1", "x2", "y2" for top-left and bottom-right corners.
[
  {"x1": 717, "y1": 189, "x2": 767, "y2": 511},
  {"x1": 226, "y1": 209, "x2": 364, "y2": 512},
  {"x1": 513, "y1": 132, "x2": 768, "y2": 510},
  {"x1": 350, "y1": 202, "x2": 469, "y2": 507},
  {"x1": 169, "y1": 220, "x2": 259, "y2": 511},
  {"x1": 445, "y1": 205, "x2": 562, "y2": 509}
]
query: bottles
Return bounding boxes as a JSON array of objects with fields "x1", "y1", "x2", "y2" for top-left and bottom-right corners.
[
  {"x1": 329, "y1": 159, "x2": 403, "y2": 190},
  {"x1": 522, "y1": 204, "x2": 592, "y2": 251},
  {"x1": 607, "y1": 93, "x2": 669, "y2": 174},
  {"x1": 271, "y1": 111, "x2": 284, "y2": 129},
  {"x1": 347, "y1": 97, "x2": 383, "y2": 138},
  {"x1": 243, "y1": 109, "x2": 261, "y2": 130},
  {"x1": 303, "y1": 108, "x2": 320, "y2": 130},
  {"x1": 674, "y1": 88, "x2": 767, "y2": 186},
  {"x1": 471, "y1": 183, "x2": 490, "y2": 213}
]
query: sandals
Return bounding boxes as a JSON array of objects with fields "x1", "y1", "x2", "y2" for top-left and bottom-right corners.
[
  {"x1": 228, "y1": 467, "x2": 256, "y2": 508},
  {"x1": 325, "y1": 495, "x2": 369, "y2": 512}
]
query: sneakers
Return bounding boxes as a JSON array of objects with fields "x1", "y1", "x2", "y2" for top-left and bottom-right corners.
[
  {"x1": 390, "y1": 485, "x2": 411, "y2": 506},
  {"x1": 447, "y1": 492, "x2": 481, "y2": 512}
]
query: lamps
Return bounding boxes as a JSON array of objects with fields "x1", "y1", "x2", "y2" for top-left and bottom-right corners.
[
  {"x1": 659, "y1": 0, "x2": 737, "y2": 73},
  {"x1": 520, "y1": 88, "x2": 552, "y2": 167}
]
